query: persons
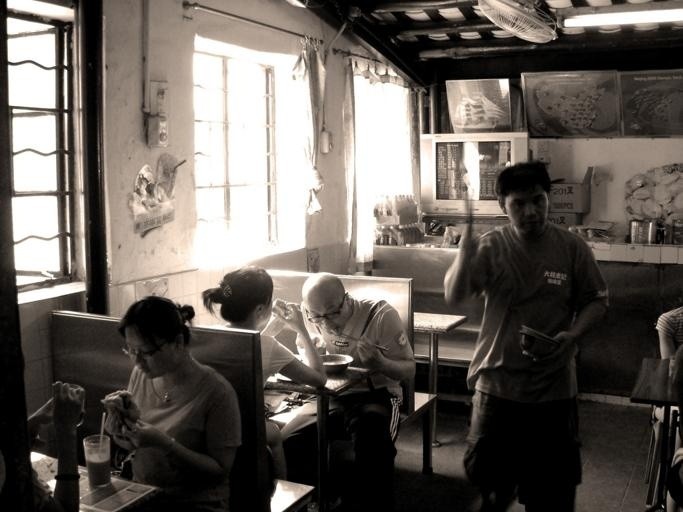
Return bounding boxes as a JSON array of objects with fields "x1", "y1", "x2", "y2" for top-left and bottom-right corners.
[
  {"x1": 443, "y1": 163, "x2": 609, "y2": 512},
  {"x1": 26, "y1": 381, "x2": 85, "y2": 512},
  {"x1": 104, "y1": 296, "x2": 242, "y2": 512},
  {"x1": 279, "y1": 273, "x2": 416, "y2": 512},
  {"x1": 200, "y1": 265, "x2": 327, "y2": 480},
  {"x1": 654, "y1": 307, "x2": 683, "y2": 512}
]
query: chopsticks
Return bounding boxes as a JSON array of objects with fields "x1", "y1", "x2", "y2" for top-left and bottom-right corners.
[{"x1": 337, "y1": 333, "x2": 390, "y2": 352}]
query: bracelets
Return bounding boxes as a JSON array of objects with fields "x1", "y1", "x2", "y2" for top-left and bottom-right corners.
[
  {"x1": 55, "y1": 472, "x2": 81, "y2": 480},
  {"x1": 153, "y1": 437, "x2": 176, "y2": 456}
]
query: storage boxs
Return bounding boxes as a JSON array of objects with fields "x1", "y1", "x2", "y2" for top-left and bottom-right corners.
[
  {"x1": 548, "y1": 166, "x2": 593, "y2": 213},
  {"x1": 547, "y1": 212, "x2": 582, "y2": 230}
]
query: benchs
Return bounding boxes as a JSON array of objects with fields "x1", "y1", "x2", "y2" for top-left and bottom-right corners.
[
  {"x1": 51, "y1": 307, "x2": 317, "y2": 508},
  {"x1": 229, "y1": 267, "x2": 440, "y2": 477}
]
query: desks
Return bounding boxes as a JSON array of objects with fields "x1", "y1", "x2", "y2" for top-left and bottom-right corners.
[
  {"x1": 260, "y1": 365, "x2": 367, "y2": 508},
  {"x1": 411, "y1": 310, "x2": 468, "y2": 475},
  {"x1": 22, "y1": 448, "x2": 157, "y2": 508}
]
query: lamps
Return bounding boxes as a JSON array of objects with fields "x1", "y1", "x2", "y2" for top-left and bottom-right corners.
[{"x1": 562, "y1": 0, "x2": 683, "y2": 28}]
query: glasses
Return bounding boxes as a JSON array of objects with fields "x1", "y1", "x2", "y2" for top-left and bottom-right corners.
[
  {"x1": 119, "y1": 340, "x2": 170, "y2": 360},
  {"x1": 304, "y1": 294, "x2": 347, "y2": 324}
]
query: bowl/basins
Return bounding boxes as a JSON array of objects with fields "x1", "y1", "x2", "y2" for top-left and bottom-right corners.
[
  {"x1": 519, "y1": 324, "x2": 561, "y2": 364},
  {"x1": 318, "y1": 353, "x2": 354, "y2": 375}
]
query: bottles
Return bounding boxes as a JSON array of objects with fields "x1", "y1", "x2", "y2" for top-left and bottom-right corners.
[
  {"x1": 384, "y1": 194, "x2": 416, "y2": 215},
  {"x1": 375, "y1": 222, "x2": 415, "y2": 245}
]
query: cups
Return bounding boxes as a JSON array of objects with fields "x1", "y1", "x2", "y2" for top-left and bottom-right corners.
[{"x1": 81, "y1": 435, "x2": 111, "y2": 489}]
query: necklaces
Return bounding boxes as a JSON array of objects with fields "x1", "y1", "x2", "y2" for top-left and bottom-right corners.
[{"x1": 156, "y1": 378, "x2": 187, "y2": 401}]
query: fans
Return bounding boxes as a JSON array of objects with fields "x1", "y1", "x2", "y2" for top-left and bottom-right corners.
[{"x1": 478, "y1": 0, "x2": 560, "y2": 44}]
